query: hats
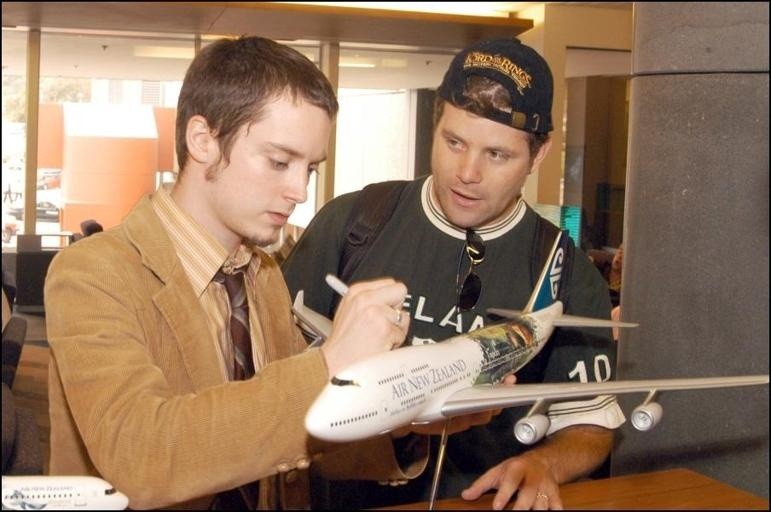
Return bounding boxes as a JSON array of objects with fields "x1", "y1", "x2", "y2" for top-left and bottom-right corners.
[{"x1": 434, "y1": 35, "x2": 558, "y2": 137}]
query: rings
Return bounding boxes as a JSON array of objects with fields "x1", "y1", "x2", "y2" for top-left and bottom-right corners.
[
  {"x1": 396, "y1": 309, "x2": 403, "y2": 324},
  {"x1": 536, "y1": 492, "x2": 548, "y2": 501}
]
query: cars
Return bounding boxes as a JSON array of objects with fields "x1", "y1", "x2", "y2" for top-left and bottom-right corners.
[{"x1": 2, "y1": 169, "x2": 62, "y2": 243}]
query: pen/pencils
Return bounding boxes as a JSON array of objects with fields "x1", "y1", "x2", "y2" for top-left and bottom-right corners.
[{"x1": 325, "y1": 274, "x2": 349, "y2": 296}]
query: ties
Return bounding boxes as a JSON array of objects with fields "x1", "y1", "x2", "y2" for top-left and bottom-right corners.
[{"x1": 205, "y1": 266, "x2": 260, "y2": 510}]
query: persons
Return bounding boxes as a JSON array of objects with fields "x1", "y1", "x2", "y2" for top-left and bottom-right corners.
[
  {"x1": 591, "y1": 240, "x2": 625, "y2": 309},
  {"x1": 273, "y1": 33, "x2": 632, "y2": 511},
  {"x1": 38, "y1": 33, "x2": 414, "y2": 511}
]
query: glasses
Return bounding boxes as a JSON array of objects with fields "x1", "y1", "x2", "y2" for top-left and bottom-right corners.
[{"x1": 452, "y1": 226, "x2": 489, "y2": 321}]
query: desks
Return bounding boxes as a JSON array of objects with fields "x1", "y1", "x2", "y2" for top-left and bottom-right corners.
[
  {"x1": 366, "y1": 462, "x2": 771, "y2": 510},
  {"x1": 2, "y1": 249, "x2": 66, "y2": 315}
]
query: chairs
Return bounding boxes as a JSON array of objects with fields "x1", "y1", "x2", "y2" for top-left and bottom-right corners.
[{"x1": 0, "y1": 268, "x2": 42, "y2": 477}]
